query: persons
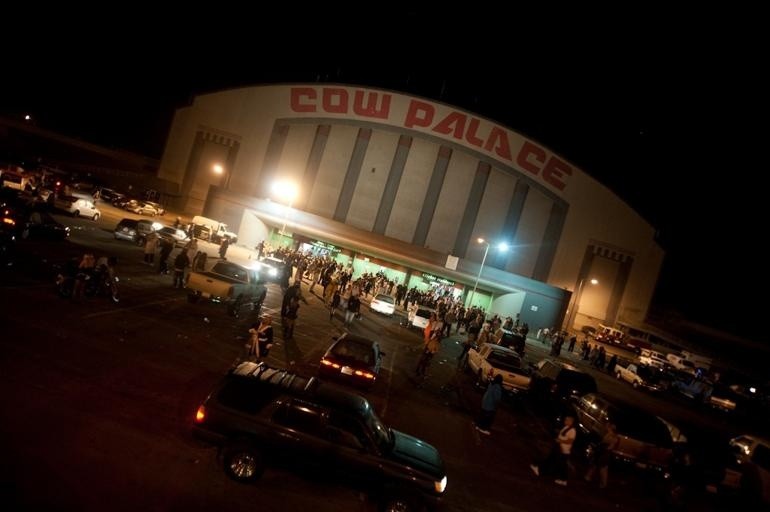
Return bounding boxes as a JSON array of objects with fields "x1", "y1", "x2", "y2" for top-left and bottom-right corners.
[
  {"x1": 80, "y1": 246, "x2": 120, "y2": 304},
  {"x1": 536, "y1": 326, "x2": 619, "y2": 374},
  {"x1": 478, "y1": 313, "x2": 529, "y2": 355},
  {"x1": 476, "y1": 374, "x2": 504, "y2": 435},
  {"x1": 247, "y1": 315, "x2": 273, "y2": 364},
  {"x1": 144, "y1": 232, "x2": 190, "y2": 290},
  {"x1": 93, "y1": 190, "x2": 100, "y2": 206},
  {"x1": 174, "y1": 216, "x2": 229, "y2": 261},
  {"x1": 529, "y1": 416, "x2": 608, "y2": 484},
  {"x1": 255, "y1": 238, "x2": 487, "y2": 378}
]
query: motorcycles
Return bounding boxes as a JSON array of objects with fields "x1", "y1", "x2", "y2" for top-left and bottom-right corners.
[{"x1": 55, "y1": 258, "x2": 120, "y2": 302}]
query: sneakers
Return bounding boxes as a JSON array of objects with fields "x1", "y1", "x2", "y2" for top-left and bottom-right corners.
[
  {"x1": 554, "y1": 479, "x2": 567, "y2": 486},
  {"x1": 531, "y1": 464, "x2": 540, "y2": 475}
]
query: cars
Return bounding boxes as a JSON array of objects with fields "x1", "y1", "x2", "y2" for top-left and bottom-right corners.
[
  {"x1": 113, "y1": 219, "x2": 267, "y2": 318},
  {"x1": 0, "y1": 196, "x2": 72, "y2": 245},
  {"x1": 534, "y1": 358, "x2": 597, "y2": 415},
  {"x1": 0, "y1": 162, "x2": 166, "y2": 220},
  {"x1": 189, "y1": 359, "x2": 447, "y2": 509},
  {"x1": 489, "y1": 325, "x2": 526, "y2": 356},
  {"x1": 463, "y1": 344, "x2": 532, "y2": 399},
  {"x1": 369, "y1": 295, "x2": 397, "y2": 317},
  {"x1": 614, "y1": 346, "x2": 720, "y2": 410},
  {"x1": 319, "y1": 331, "x2": 387, "y2": 389},
  {"x1": 730, "y1": 436, "x2": 764, "y2": 475},
  {"x1": 571, "y1": 393, "x2": 729, "y2": 486},
  {"x1": 404, "y1": 304, "x2": 437, "y2": 332}
]
